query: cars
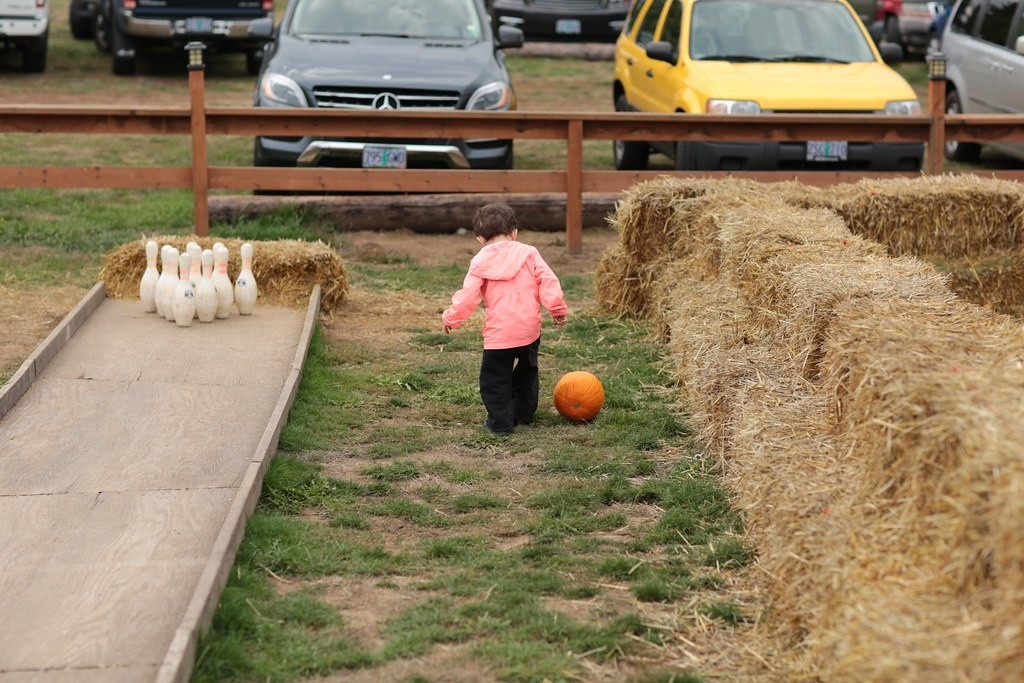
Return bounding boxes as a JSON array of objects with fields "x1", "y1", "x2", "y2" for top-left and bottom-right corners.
[
  {"x1": 252, "y1": 0, "x2": 526, "y2": 196},
  {"x1": 901, "y1": 2, "x2": 945, "y2": 54},
  {"x1": 941, "y1": 0, "x2": 1024, "y2": 163},
  {"x1": 0, "y1": 0, "x2": 50, "y2": 73},
  {"x1": 612, "y1": 0, "x2": 924, "y2": 173}
]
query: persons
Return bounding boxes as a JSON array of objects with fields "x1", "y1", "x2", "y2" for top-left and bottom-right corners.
[{"x1": 441, "y1": 202, "x2": 568, "y2": 434}]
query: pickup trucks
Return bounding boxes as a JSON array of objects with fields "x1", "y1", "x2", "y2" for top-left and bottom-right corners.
[{"x1": 91, "y1": 0, "x2": 274, "y2": 76}]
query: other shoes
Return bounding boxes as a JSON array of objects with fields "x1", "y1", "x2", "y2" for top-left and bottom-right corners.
[{"x1": 483, "y1": 421, "x2": 511, "y2": 435}]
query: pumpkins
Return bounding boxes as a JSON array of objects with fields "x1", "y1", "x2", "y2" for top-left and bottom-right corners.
[{"x1": 553, "y1": 371, "x2": 604, "y2": 421}]
